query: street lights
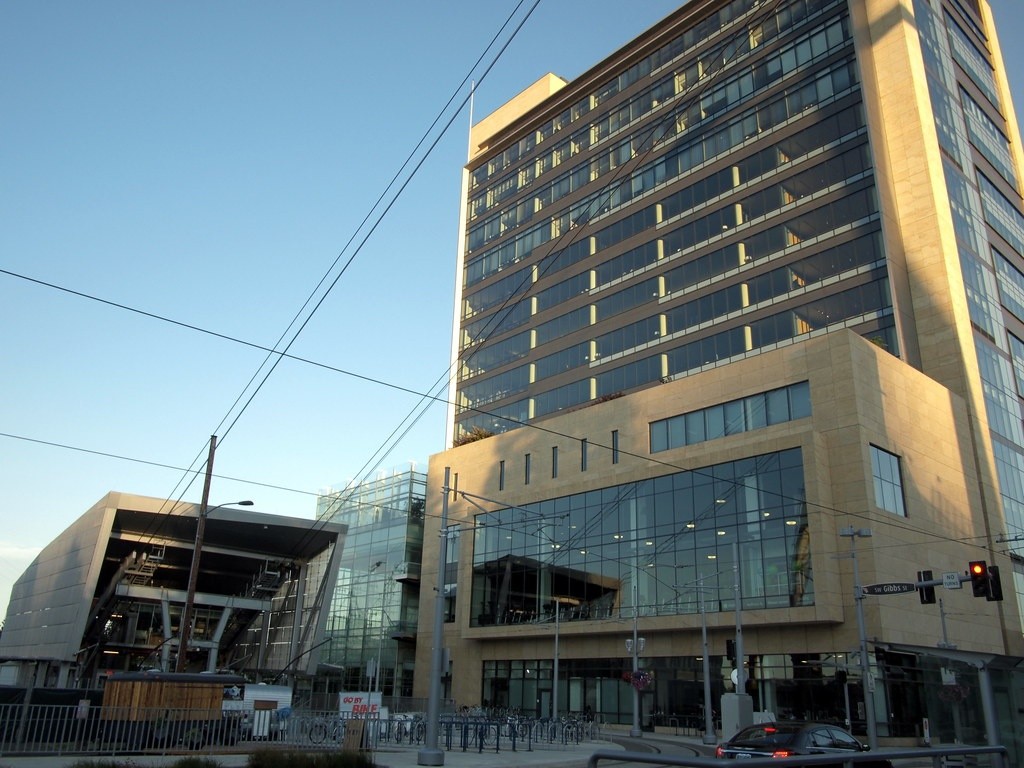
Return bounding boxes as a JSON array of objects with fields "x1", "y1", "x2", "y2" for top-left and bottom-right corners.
[
  {"x1": 175, "y1": 500, "x2": 256, "y2": 674},
  {"x1": 839, "y1": 526, "x2": 878, "y2": 752}
]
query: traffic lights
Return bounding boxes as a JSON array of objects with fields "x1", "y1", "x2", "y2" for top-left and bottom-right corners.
[{"x1": 969, "y1": 560, "x2": 992, "y2": 597}]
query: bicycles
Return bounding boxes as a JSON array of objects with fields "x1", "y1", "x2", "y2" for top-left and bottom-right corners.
[
  {"x1": 308, "y1": 721, "x2": 347, "y2": 744},
  {"x1": 466, "y1": 716, "x2": 497, "y2": 746},
  {"x1": 395, "y1": 714, "x2": 424, "y2": 742},
  {"x1": 502, "y1": 719, "x2": 528, "y2": 738}
]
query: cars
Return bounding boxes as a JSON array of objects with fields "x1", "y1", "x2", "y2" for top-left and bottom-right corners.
[{"x1": 716, "y1": 721, "x2": 894, "y2": 768}]
